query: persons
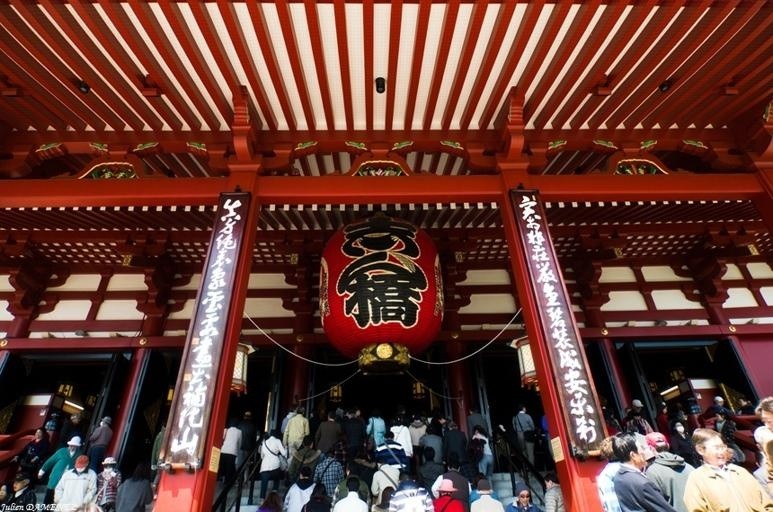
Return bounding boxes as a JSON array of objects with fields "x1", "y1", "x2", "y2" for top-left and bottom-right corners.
[{"x1": 0, "y1": 391, "x2": 773, "y2": 511}]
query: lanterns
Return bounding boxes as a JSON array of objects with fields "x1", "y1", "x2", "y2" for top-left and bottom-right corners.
[
  {"x1": 165, "y1": 385, "x2": 175, "y2": 408},
  {"x1": 328, "y1": 383, "x2": 343, "y2": 404},
  {"x1": 57, "y1": 382, "x2": 73, "y2": 398},
  {"x1": 230, "y1": 342, "x2": 256, "y2": 398},
  {"x1": 411, "y1": 381, "x2": 425, "y2": 400}
]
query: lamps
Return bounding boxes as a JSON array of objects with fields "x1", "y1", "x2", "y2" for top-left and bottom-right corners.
[
  {"x1": 228, "y1": 344, "x2": 254, "y2": 397},
  {"x1": 80, "y1": 82, "x2": 89, "y2": 92},
  {"x1": 374, "y1": 77, "x2": 386, "y2": 94},
  {"x1": 661, "y1": 81, "x2": 669, "y2": 92}
]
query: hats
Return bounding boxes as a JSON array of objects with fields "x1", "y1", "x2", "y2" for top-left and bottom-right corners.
[
  {"x1": 67, "y1": 436, "x2": 81, "y2": 446},
  {"x1": 15, "y1": 472, "x2": 29, "y2": 482},
  {"x1": 437, "y1": 479, "x2": 458, "y2": 492},
  {"x1": 713, "y1": 396, "x2": 723, "y2": 401},
  {"x1": 102, "y1": 416, "x2": 111, "y2": 424},
  {"x1": 633, "y1": 399, "x2": 643, "y2": 407},
  {"x1": 647, "y1": 432, "x2": 667, "y2": 446},
  {"x1": 477, "y1": 479, "x2": 490, "y2": 489},
  {"x1": 101, "y1": 457, "x2": 117, "y2": 465},
  {"x1": 515, "y1": 482, "x2": 530, "y2": 495},
  {"x1": 75, "y1": 455, "x2": 89, "y2": 468}
]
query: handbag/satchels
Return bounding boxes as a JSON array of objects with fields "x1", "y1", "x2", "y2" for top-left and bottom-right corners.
[
  {"x1": 364, "y1": 437, "x2": 375, "y2": 452},
  {"x1": 523, "y1": 430, "x2": 536, "y2": 443},
  {"x1": 279, "y1": 454, "x2": 289, "y2": 473},
  {"x1": 470, "y1": 438, "x2": 483, "y2": 465}
]
